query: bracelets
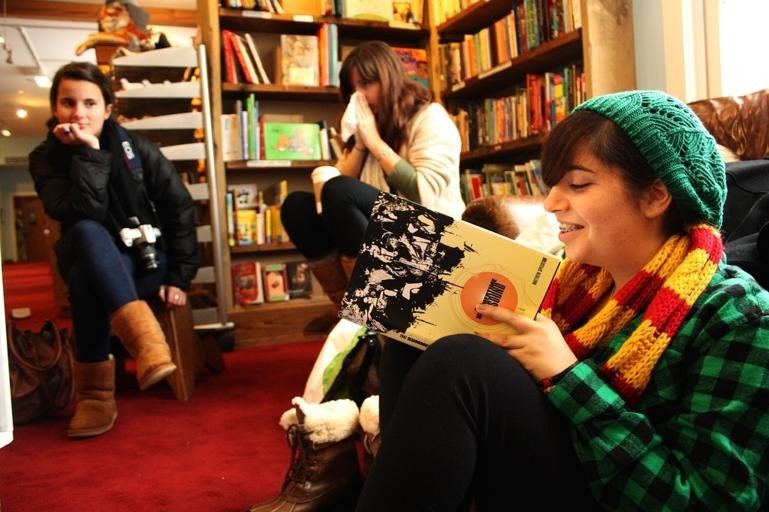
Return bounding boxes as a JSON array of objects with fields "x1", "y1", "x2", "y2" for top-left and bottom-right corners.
[{"x1": 375, "y1": 146, "x2": 393, "y2": 162}]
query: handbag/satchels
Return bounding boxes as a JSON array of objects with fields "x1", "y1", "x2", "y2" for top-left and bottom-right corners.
[{"x1": 5, "y1": 317, "x2": 77, "y2": 427}]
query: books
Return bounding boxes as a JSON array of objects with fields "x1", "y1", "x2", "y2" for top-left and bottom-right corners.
[
  {"x1": 449, "y1": 65, "x2": 585, "y2": 151},
  {"x1": 223, "y1": 30, "x2": 272, "y2": 85},
  {"x1": 227, "y1": 180, "x2": 289, "y2": 245},
  {"x1": 231, "y1": 260, "x2": 264, "y2": 305},
  {"x1": 263, "y1": 264, "x2": 289, "y2": 303},
  {"x1": 220, "y1": 0, "x2": 427, "y2": 31},
  {"x1": 432, "y1": 0, "x2": 582, "y2": 89},
  {"x1": 222, "y1": 94, "x2": 346, "y2": 163},
  {"x1": 392, "y1": 46, "x2": 430, "y2": 94},
  {"x1": 339, "y1": 191, "x2": 561, "y2": 350},
  {"x1": 275, "y1": 35, "x2": 320, "y2": 86},
  {"x1": 460, "y1": 159, "x2": 551, "y2": 204},
  {"x1": 287, "y1": 260, "x2": 312, "y2": 299}
]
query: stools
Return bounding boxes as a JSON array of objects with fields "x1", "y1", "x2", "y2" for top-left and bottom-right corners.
[{"x1": 77, "y1": 297, "x2": 197, "y2": 405}]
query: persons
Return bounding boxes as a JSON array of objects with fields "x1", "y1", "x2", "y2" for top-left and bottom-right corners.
[
  {"x1": 30, "y1": 61, "x2": 203, "y2": 437},
  {"x1": 356, "y1": 91, "x2": 769, "y2": 512},
  {"x1": 281, "y1": 41, "x2": 465, "y2": 336}
]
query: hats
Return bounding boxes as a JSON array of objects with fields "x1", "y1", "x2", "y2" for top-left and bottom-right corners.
[{"x1": 570, "y1": 89, "x2": 728, "y2": 230}]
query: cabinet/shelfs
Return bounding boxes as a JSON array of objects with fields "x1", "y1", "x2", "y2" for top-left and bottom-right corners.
[
  {"x1": 431, "y1": 0, "x2": 635, "y2": 220},
  {"x1": 201, "y1": 2, "x2": 433, "y2": 343}
]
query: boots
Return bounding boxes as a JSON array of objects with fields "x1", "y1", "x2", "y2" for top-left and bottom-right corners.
[
  {"x1": 109, "y1": 299, "x2": 177, "y2": 391},
  {"x1": 65, "y1": 354, "x2": 119, "y2": 438},
  {"x1": 359, "y1": 394, "x2": 382, "y2": 460},
  {"x1": 246, "y1": 395, "x2": 360, "y2": 512},
  {"x1": 301, "y1": 250, "x2": 348, "y2": 336}
]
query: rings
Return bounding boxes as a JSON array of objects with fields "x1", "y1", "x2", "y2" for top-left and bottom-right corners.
[
  {"x1": 173, "y1": 292, "x2": 180, "y2": 302},
  {"x1": 64, "y1": 128, "x2": 73, "y2": 135}
]
